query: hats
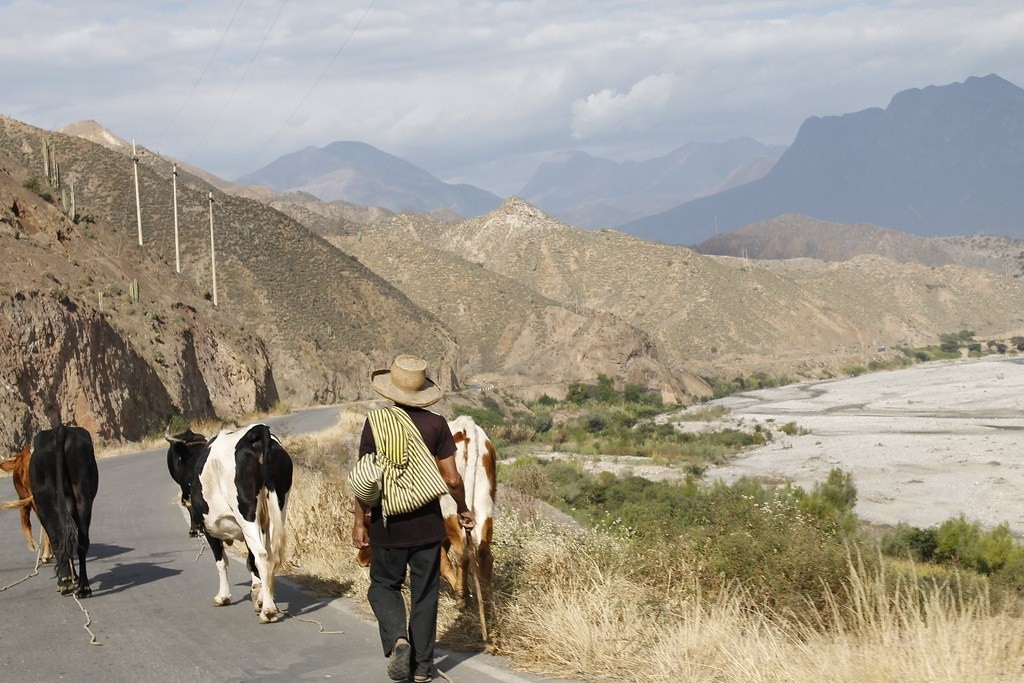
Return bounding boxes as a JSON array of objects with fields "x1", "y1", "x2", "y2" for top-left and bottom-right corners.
[{"x1": 369, "y1": 355, "x2": 442, "y2": 408}]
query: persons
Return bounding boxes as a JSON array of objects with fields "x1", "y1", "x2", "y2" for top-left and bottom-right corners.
[
  {"x1": 483, "y1": 377, "x2": 485, "y2": 384},
  {"x1": 351, "y1": 355, "x2": 476, "y2": 683}
]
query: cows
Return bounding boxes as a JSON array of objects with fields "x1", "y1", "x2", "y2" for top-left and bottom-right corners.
[
  {"x1": 438, "y1": 414, "x2": 497, "y2": 611},
  {"x1": 0, "y1": 445, "x2": 53, "y2": 563},
  {"x1": 29, "y1": 423, "x2": 99, "y2": 599},
  {"x1": 163, "y1": 423, "x2": 294, "y2": 623}
]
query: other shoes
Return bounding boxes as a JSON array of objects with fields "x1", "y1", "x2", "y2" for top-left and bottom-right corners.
[
  {"x1": 388, "y1": 642, "x2": 411, "y2": 682},
  {"x1": 409, "y1": 674, "x2": 432, "y2": 683}
]
